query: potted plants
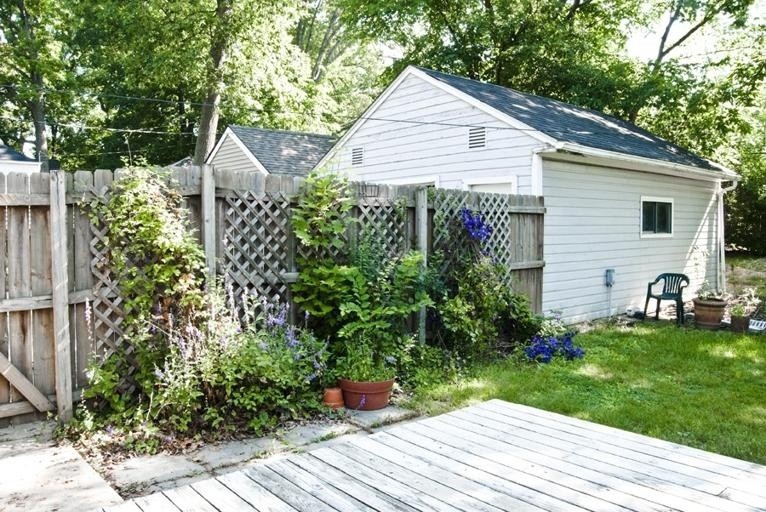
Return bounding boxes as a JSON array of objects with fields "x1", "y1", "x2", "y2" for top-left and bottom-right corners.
[
  {"x1": 728, "y1": 304, "x2": 750, "y2": 333},
  {"x1": 690, "y1": 280, "x2": 728, "y2": 331},
  {"x1": 340, "y1": 355, "x2": 397, "y2": 411}
]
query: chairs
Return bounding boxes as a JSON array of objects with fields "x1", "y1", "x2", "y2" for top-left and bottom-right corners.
[{"x1": 642, "y1": 270, "x2": 690, "y2": 327}]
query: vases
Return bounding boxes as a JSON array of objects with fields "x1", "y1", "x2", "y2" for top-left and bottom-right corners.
[{"x1": 321, "y1": 386, "x2": 344, "y2": 411}]
